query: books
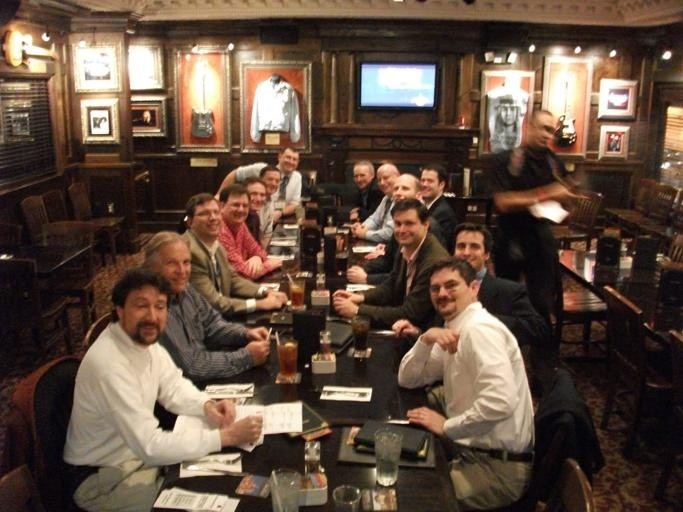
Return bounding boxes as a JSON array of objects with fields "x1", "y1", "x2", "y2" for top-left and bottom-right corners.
[{"x1": 353, "y1": 420, "x2": 429, "y2": 460}]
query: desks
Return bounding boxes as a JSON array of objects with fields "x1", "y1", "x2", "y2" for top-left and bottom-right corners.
[
  {"x1": 149, "y1": 203, "x2": 463, "y2": 512},
  {"x1": 551, "y1": 246, "x2": 683, "y2": 357}
]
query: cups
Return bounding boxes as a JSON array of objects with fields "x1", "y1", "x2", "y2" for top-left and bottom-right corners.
[
  {"x1": 268, "y1": 468, "x2": 301, "y2": 512},
  {"x1": 374, "y1": 427, "x2": 403, "y2": 486},
  {"x1": 351, "y1": 316, "x2": 369, "y2": 351},
  {"x1": 288, "y1": 280, "x2": 305, "y2": 309},
  {"x1": 277, "y1": 340, "x2": 298, "y2": 379},
  {"x1": 294, "y1": 207, "x2": 305, "y2": 224},
  {"x1": 333, "y1": 485, "x2": 362, "y2": 512},
  {"x1": 597, "y1": 237, "x2": 622, "y2": 267}
]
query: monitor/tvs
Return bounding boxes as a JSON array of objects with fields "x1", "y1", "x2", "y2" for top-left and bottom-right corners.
[{"x1": 357, "y1": 60, "x2": 439, "y2": 110}]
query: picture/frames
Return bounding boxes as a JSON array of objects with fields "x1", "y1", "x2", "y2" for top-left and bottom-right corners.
[
  {"x1": 478, "y1": 69, "x2": 536, "y2": 156},
  {"x1": 539, "y1": 55, "x2": 595, "y2": 158},
  {"x1": 238, "y1": 60, "x2": 313, "y2": 154},
  {"x1": 598, "y1": 125, "x2": 630, "y2": 159},
  {"x1": 131, "y1": 97, "x2": 170, "y2": 139},
  {"x1": 4, "y1": 106, "x2": 36, "y2": 143},
  {"x1": 171, "y1": 49, "x2": 232, "y2": 152},
  {"x1": 596, "y1": 79, "x2": 639, "y2": 120},
  {"x1": 71, "y1": 42, "x2": 121, "y2": 93},
  {"x1": 128, "y1": 44, "x2": 168, "y2": 93},
  {"x1": 80, "y1": 96, "x2": 119, "y2": 145}
]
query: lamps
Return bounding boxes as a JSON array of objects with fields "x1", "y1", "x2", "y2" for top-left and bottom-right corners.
[
  {"x1": 124, "y1": 12, "x2": 143, "y2": 34},
  {"x1": 40, "y1": 21, "x2": 51, "y2": 43}
]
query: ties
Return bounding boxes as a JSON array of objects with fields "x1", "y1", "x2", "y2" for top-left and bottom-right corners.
[{"x1": 280, "y1": 176, "x2": 288, "y2": 199}]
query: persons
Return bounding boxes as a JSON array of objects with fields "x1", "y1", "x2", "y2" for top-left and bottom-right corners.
[
  {"x1": 392, "y1": 222, "x2": 550, "y2": 417},
  {"x1": 213, "y1": 147, "x2": 303, "y2": 281},
  {"x1": 178, "y1": 193, "x2": 287, "y2": 317},
  {"x1": 249, "y1": 74, "x2": 302, "y2": 145},
  {"x1": 136, "y1": 230, "x2": 270, "y2": 383},
  {"x1": 397, "y1": 259, "x2": 537, "y2": 511},
  {"x1": 480, "y1": 111, "x2": 590, "y2": 332},
  {"x1": 490, "y1": 95, "x2": 522, "y2": 154},
  {"x1": 63, "y1": 269, "x2": 263, "y2": 511},
  {"x1": 332, "y1": 199, "x2": 452, "y2": 332},
  {"x1": 336, "y1": 160, "x2": 457, "y2": 287}
]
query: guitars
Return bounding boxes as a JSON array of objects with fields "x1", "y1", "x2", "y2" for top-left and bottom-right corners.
[
  {"x1": 191, "y1": 59, "x2": 215, "y2": 138},
  {"x1": 553, "y1": 81, "x2": 577, "y2": 146},
  {"x1": 4, "y1": 31, "x2": 55, "y2": 66}
]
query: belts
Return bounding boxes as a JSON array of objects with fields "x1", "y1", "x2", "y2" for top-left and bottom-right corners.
[{"x1": 469, "y1": 446, "x2": 533, "y2": 461}]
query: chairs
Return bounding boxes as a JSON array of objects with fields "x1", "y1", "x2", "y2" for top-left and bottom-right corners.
[
  {"x1": 536, "y1": 456, "x2": 596, "y2": 512},
  {"x1": 445, "y1": 194, "x2": 492, "y2": 223},
  {"x1": 604, "y1": 282, "x2": 683, "y2": 501},
  {"x1": 517, "y1": 218, "x2": 610, "y2": 355},
  {"x1": 519, "y1": 367, "x2": 604, "y2": 500},
  {"x1": 553, "y1": 190, "x2": 605, "y2": 249},
  {"x1": 1, "y1": 181, "x2": 121, "y2": 364},
  {"x1": 310, "y1": 183, "x2": 354, "y2": 204},
  {"x1": 26, "y1": 356, "x2": 81, "y2": 512},
  {"x1": 604, "y1": 177, "x2": 683, "y2": 286}
]
